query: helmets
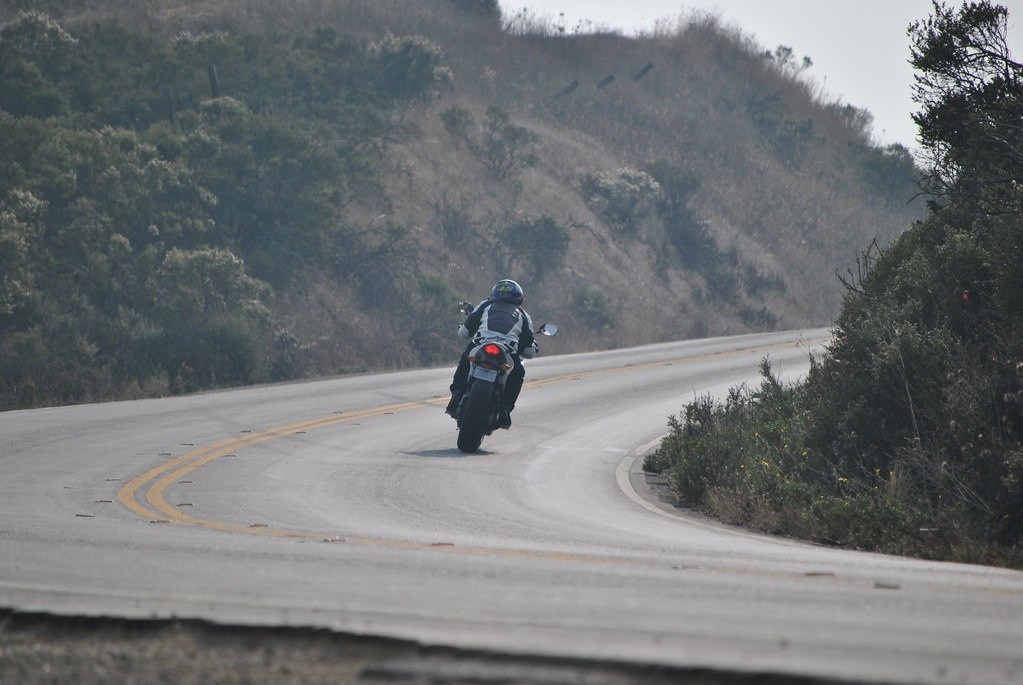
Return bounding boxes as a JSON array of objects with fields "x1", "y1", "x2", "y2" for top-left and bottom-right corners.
[{"x1": 491, "y1": 279, "x2": 524, "y2": 305}]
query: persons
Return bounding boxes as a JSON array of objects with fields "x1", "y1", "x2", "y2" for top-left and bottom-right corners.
[{"x1": 446, "y1": 279, "x2": 538, "y2": 430}]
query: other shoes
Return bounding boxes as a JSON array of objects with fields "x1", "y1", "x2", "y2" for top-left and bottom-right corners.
[
  {"x1": 447, "y1": 387, "x2": 462, "y2": 410},
  {"x1": 500, "y1": 408, "x2": 512, "y2": 429}
]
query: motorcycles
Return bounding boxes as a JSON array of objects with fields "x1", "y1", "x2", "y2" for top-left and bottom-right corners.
[{"x1": 446, "y1": 300, "x2": 558, "y2": 453}]
